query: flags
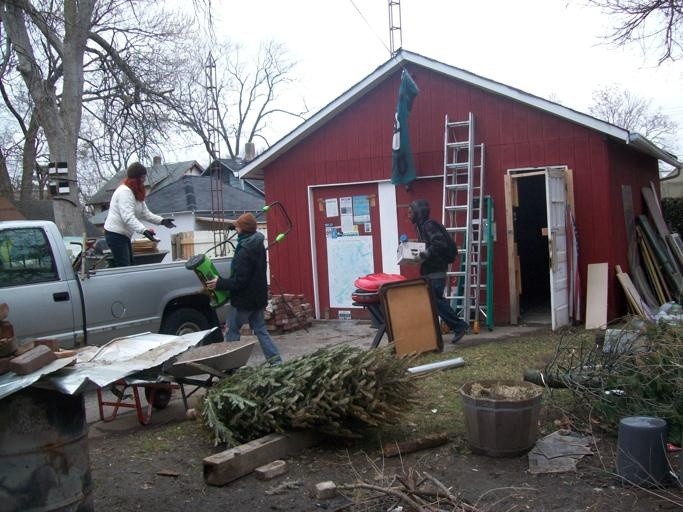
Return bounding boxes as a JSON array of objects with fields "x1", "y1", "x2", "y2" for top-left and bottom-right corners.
[{"x1": 388, "y1": 70, "x2": 418, "y2": 186}]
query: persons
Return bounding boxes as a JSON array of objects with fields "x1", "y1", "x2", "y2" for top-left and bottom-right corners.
[
  {"x1": 408, "y1": 199, "x2": 468, "y2": 343},
  {"x1": 104, "y1": 162, "x2": 177, "y2": 268},
  {"x1": 206, "y1": 212, "x2": 282, "y2": 365}
]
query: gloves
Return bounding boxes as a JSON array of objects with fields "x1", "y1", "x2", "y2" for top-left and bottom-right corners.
[
  {"x1": 162, "y1": 218, "x2": 177, "y2": 228},
  {"x1": 144, "y1": 230, "x2": 160, "y2": 242}
]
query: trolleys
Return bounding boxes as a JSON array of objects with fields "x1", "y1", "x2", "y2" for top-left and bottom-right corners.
[
  {"x1": 185, "y1": 202, "x2": 293, "y2": 310},
  {"x1": 146, "y1": 338, "x2": 256, "y2": 411}
]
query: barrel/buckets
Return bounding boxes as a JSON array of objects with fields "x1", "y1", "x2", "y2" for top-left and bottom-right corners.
[
  {"x1": 0, "y1": 384, "x2": 94, "y2": 511},
  {"x1": 616, "y1": 416, "x2": 675, "y2": 488}
]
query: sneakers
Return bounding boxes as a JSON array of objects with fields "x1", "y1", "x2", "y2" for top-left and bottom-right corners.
[{"x1": 452, "y1": 328, "x2": 466, "y2": 344}]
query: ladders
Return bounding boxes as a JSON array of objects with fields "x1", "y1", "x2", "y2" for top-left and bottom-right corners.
[{"x1": 441, "y1": 112, "x2": 494, "y2": 334}]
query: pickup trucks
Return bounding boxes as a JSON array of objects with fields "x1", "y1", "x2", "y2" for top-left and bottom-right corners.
[{"x1": 0, "y1": 219, "x2": 237, "y2": 351}]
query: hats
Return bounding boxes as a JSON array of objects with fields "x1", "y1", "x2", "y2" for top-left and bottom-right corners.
[
  {"x1": 233, "y1": 213, "x2": 256, "y2": 232},
  {"x1": 128, "y1": 162, "x2": 146, "y2": 176}
]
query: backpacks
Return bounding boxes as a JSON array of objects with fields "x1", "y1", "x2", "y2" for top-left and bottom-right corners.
[{"x1": 422, "y1": 220, "x2": 456, "y2": 264}]
